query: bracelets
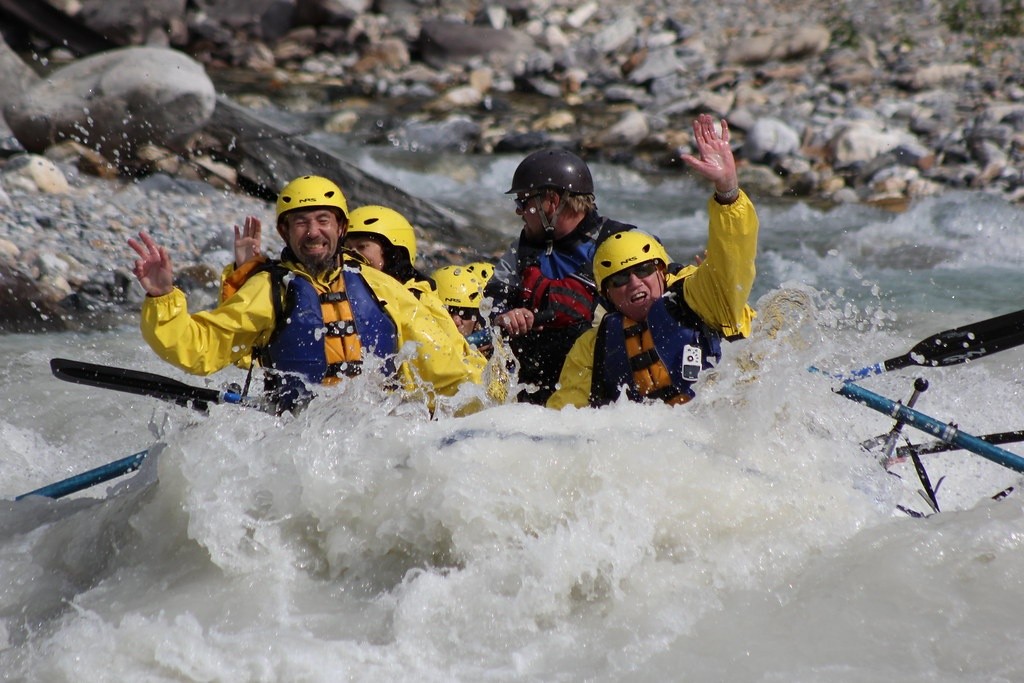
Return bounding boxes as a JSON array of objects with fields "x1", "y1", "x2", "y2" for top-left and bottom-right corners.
[{"x1": 714, "y1": 184, "x2": 739, "y2": 199}]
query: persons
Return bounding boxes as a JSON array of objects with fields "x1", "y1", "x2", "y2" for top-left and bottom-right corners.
[
  {"x1": 127, "y1": 175, "x2": 510, "y2": 421},
  {"x1": 546, "y1": 113, "x2": 760, "y2": 411},
  {"x1": 483, "y1": 149, "x2": 663, "y2": 408}
]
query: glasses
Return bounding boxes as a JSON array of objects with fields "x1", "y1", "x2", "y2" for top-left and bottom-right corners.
[
  {"x1": 514, "y1": 192, "x2": 546, "y2": 213},
  {"x1": 609, "y1": 259, "x2": 659, "y2": 288},
  {"x1": 448, "y1": 306, "x2": 479, "y2": 321}
]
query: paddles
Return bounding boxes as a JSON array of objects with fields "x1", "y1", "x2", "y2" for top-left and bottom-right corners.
[
  {"x1": 14, "y1": 327, "x2": 507, "y2": 506},
  {"x1": 810, "y1": 365, "x2": 1024, "y2": 474},
  {"x1": 827, "y1": 308, "x2": 1024, "y2": 382},
  {"x1": 48, "y1": 357, "x2": 278, "y2": 411}
]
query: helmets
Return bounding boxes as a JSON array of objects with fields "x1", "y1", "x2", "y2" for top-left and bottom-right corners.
[
  {"x1": 591, "y1": 231, "x2": 669, "y2": 301},
  {"x1": 273, "y1": 175, "x2": 349, "y2": 236},
  {"x1": 428, "y1": 265, "x2": 483, "y2": 308},
  {"x1": 466, "y1": 262, "x2": 497, "y2": 291},
  {"x1": 345, "y1": 205, "x2": 416, "y2": 270},
  {"x1": 503, "y1": 148, "x2": 594, "y2": 194}
]
